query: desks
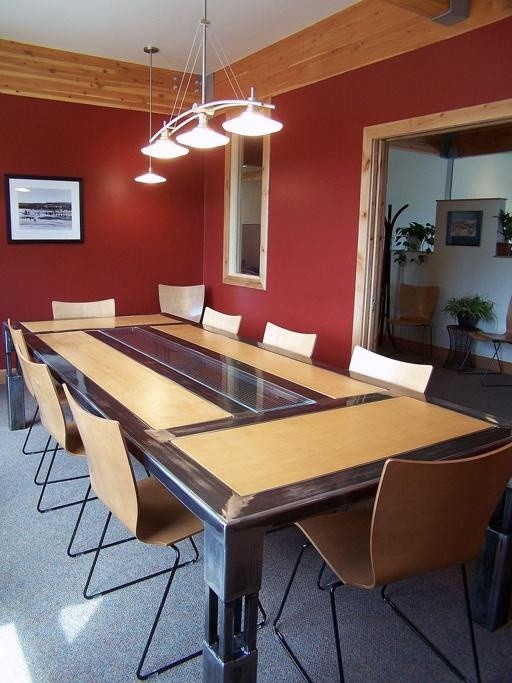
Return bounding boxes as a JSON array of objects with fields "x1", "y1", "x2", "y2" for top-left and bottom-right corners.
[{"x1": 2, "y1": 312, "x2": 511, "y2": 682}]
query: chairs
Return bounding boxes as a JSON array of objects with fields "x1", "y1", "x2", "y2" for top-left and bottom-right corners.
[
  {"x1": 200, "y1": 306, "x2": 243, "y2": 334},
  {"x1": 14, "y1": 345, "x2": 143, "y2": 558},
  {"x1": 51, "y1": 296, "x2": 116, "y2": 320},
  {"x1": 385, "y1": 284, "x2": 442, "y2": 358},
  {"x1": 271, "y1": 444, "x2": 512, "y2": 682},
  {"x1": 6, "y1": 320, "x2": 90, "y2": 486},
  {"x1": 456, "y1": 295, "x2": 512, "y2": 387},
  {"x1": 348, "y1": 346, "x2": 433, "y2": 397},
  {"x1": 158, "y1": 283, "x2": 206, "y2": 324},
  {"x1": 61, "y1": 382, "x2": 268, "y2": 680},
  {"x1": 261, "y1": 321, "x2": 318, "y2": 358}
]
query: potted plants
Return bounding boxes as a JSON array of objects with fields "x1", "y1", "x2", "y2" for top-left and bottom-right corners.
[
  {"x1": 442, "y1": 295, "x2": 499, "y2": 325},
  {"x1": 393, "y1": 221, "x2": 435, "y2": 267},
  {"x1": 492, "y1": 208, "x2": 512, "y2": 257}
]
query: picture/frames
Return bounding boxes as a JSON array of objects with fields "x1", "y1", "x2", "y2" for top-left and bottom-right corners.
[
  {"x1": 444, "y1": 210, "x2": 484, "y2": 247},
  {"x1": 6, "y1": 174, "x2": 84, "y2": 243}
]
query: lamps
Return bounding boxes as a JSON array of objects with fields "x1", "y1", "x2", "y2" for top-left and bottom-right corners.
[
  {"x1": 140, "y1": 0, "x2": 284, "y2": 159},
  {"x1": 134, "y1": 46, "x2": 168, "y2": 186}
]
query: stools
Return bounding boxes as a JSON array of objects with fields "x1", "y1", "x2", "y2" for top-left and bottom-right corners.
[{"x1": 442, "y1": 325, "x2": 480, "y2": 372}]
